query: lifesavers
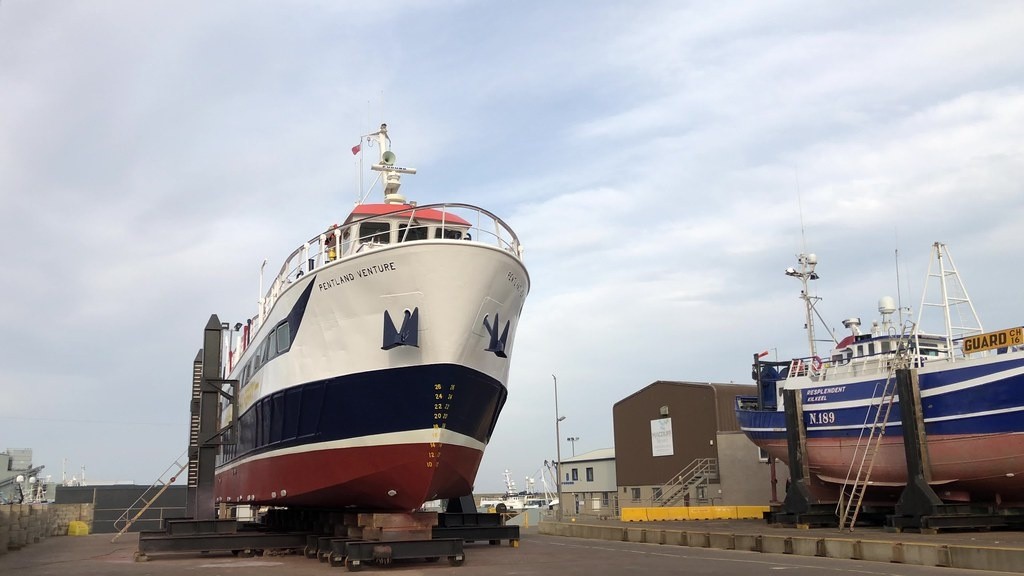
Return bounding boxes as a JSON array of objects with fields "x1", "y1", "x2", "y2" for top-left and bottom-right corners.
[
  {"x1": 792, "y1": 360, "x2": 802, "y2": 371},
  {"x1": 812, "y1": 355, "x2": 822, "y2": 371}
]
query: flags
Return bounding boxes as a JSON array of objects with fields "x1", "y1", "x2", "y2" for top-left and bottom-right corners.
[{"x1": 352, "y1": 145, "x2": 359, "y2": 155}]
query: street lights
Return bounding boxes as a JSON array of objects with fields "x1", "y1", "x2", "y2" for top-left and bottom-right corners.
[
  {"x1": 567, "y1": 436, "x2": 579, "y2": 456},
  {"x1": 551, "y1": 374, "x2": 566, "y2": 520}
]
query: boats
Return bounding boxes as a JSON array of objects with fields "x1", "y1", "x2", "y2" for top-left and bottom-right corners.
[
  {"x1": 733, "y1": 239, "x2": 1024, "y2": 505},
  {"x1": 214, "y1": 122, "x2": 531, "y2": 508}
]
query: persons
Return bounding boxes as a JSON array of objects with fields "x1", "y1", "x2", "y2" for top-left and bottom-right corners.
[{"x1": 328, "y1": 234, "x2": 336, "y2": 262}]
